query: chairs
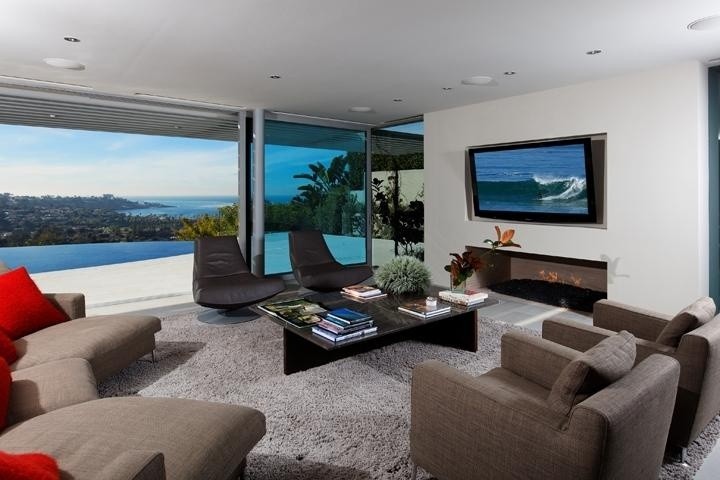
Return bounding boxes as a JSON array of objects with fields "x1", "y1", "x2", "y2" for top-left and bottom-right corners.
[
  {"x1": 410, "y1": 330, "x2": 681, "y2": 480},
  {"x1": 191, "y1": 235, "x2": 287, "y2": 317},
  {"x1": 543, "y1": 298, "x2": 720, "y2": 464},
  {"x1": 289, "y1": 229, "x2": 374, "y2": 292}
]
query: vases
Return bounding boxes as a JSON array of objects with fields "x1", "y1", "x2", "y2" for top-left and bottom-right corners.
[{"x1": 450, "y1": 274, "x2": 466, "y2": 293}]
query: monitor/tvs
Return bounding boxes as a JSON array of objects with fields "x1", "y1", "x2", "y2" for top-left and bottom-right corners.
[{"x1": 468, "y1": 137, "x2": 597, "y2": 223}]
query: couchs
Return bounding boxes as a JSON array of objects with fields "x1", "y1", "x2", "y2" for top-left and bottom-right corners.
[{"x1": 0, "y1": 260, "x2": 265, "y2": 480}]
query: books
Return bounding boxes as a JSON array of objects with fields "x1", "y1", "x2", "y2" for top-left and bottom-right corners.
[{"x1": 257, "y1": 283, "x2": 489, "y2": 342}]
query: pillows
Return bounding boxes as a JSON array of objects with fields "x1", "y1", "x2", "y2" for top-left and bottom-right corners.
[
  {"x1": 0, "y1": 448, "x2": 61, "y2": 480},
  {"x1": 0, "y1": 356, "x2": 12, "y2": 432},
  {"x1": 0, "y1": 266, "x2": 70, "y2": 340},
  {"x1": 0, "y1": 330, "x2": 18, "y2": 365},
  {"x1": 548, "y1": 330, "x2": 636, "y2": 416},
  {"x1": 656, "y1": 297, "x2": 716, "y2": 347}
]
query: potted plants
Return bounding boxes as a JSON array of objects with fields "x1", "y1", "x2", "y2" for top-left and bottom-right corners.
[{"x1": 375, "y1": 254, "x2": 432, "y2": 300}]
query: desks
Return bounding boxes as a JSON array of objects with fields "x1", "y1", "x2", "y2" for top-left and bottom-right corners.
[{"x1": 248, "y1": 281, "x2": 502, "y2": 376}]
